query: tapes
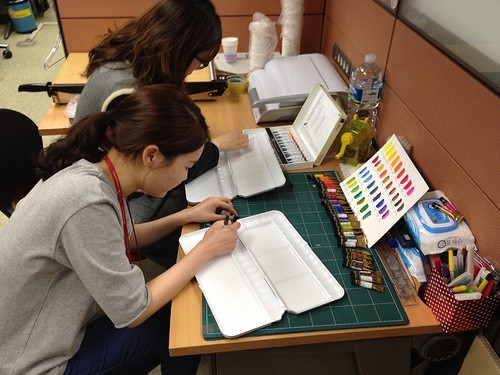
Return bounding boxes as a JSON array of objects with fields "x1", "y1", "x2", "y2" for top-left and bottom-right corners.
[{"x1": 226, "y1": 75, "x2": 247, "y2": 93}]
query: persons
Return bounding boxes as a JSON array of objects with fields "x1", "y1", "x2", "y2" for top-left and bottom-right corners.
[
  {"x1": 72, "y1": 0, "x2": 249, "y2": 268},
  {"x1": 0, "y1": 83, "x2": 241, "y2": 375}
]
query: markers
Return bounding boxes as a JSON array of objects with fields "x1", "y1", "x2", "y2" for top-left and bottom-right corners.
[{"x1": 432, "y1": 244, "x2": 492, "y2": 300}]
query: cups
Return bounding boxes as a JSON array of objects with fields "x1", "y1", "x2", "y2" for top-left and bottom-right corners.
[
  {"x1": 250, "y1": 22, "x2": 268, "y2": 70},
  {"x1": 281, "y1": 0, "x2": 304, "y2": 55},
  {"x1": 222, "y1": 37, "x2": 238, "y2": 63}
]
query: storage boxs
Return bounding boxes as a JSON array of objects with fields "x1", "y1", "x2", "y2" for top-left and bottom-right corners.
[{"x1": 423, "y1": 265, "x2": 500, "y2": 334}]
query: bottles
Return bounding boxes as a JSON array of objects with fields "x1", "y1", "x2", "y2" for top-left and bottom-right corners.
[{"x1": 348, "y1": 53, "x2": 384, "y2": 148}]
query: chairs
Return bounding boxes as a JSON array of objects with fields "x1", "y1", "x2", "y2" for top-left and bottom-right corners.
[{"x1": 0, "y1": 108, "x2": 46, "y2": 218}]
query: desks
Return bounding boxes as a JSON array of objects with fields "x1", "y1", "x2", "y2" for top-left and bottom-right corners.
[{"x1": 36, "y1": 51, "x2": 483, "y2": 375}]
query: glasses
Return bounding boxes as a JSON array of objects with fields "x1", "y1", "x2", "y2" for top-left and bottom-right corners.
[{"x1": 192, "y1": 55, "x2": 208, "y2": 69}]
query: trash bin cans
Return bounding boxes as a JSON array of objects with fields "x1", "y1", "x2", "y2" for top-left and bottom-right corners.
[{"x1": 4, "y1": 0, "x2": 37, "y2": 33}]
